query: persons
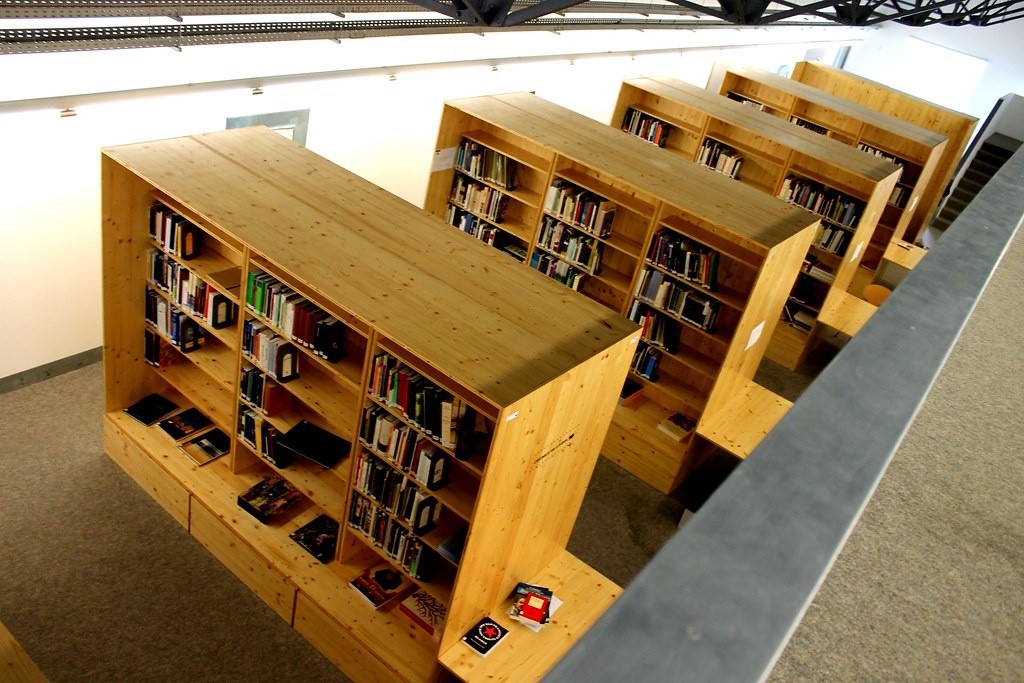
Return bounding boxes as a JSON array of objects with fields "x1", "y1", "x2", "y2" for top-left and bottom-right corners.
[{"x1": 511, "y1": 595, "x2": 526, "y2": 616}]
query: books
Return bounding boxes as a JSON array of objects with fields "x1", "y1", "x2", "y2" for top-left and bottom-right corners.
[
  {"x1": 657, "y1": 411, "x2": 698, "y2": 441},
  {"x1": 779, "y1": 174, "x2": 864, "y2": 255},
  {"x1": 122, "y1": 203, "x2": 494, "y2": 637},
  {"x1": 506, "y1": 582, "x2": 563, "y2": 633},
  {"x1": 726, "y1": 93, "x2": 769, "y2": 113},
  {"x1": 791, "y1": 117, "x2": 834, "y2": 137},
  {"x1": 461, "y1": 617, "x2": 510, "y2": 658},
  {"x1": 780, "y1": 252, "x2": 837, "y2": 335},
  {"x1": 621, "y1": 109, "x2": 672, "y2": 149},
  {"x1": 698, "y1": 138, "x2": 746, "y2": 179},
  {"x1": 857, "y1": 143, "x2": 908, "y2": 208},
  {"x1": 614, "y1": 228, "x2": 722, "y2": 405},
  {"x1": 443, "y1": 140, "x2": 618, "y2": 292}
]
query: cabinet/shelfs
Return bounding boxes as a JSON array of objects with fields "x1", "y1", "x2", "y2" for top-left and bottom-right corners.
[
  {"x1": 705, "y1": 59, "x2": 950, "y2": 301},
  {"x1": 610, "y1": 72, "x2": 902, "y2": 371},
  {"x1": 99, "y1": 126, "x2": 626, "y2": 683},
  {"x1": 791, "y1": 60, "x2": 980, "y2": 250},
  {"x1": 423, "y1": 89, "x2": 821, "y2": 493}
]
